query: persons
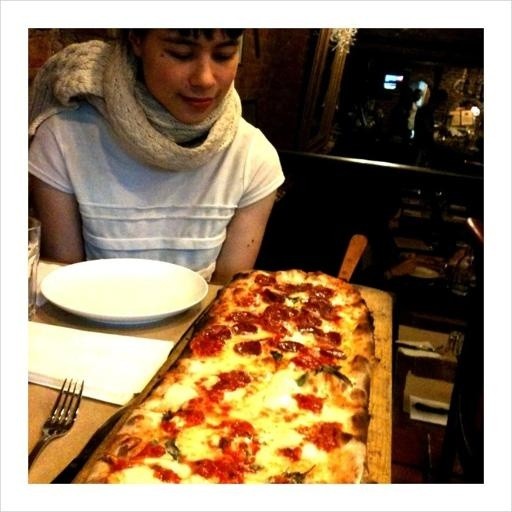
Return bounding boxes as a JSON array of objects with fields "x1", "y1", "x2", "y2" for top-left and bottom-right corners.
[
  {"x1": 28, "y1": 29, "x2": 289, "y2": 285},
  {"x1": 413, "y1": 89, "x2": 449, "y2": 166},
  {"x1": 376, "y1": 197, "x2": 417, "y2": 284}
]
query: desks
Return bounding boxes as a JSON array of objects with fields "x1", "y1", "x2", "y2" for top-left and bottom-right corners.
[{"x1": 28, "y1": 259, "x2": 394, "y2": 482}]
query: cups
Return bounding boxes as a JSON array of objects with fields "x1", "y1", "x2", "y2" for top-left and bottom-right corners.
[{"x1": 29, "y1": 215, "x2": 44, "y2": 323}]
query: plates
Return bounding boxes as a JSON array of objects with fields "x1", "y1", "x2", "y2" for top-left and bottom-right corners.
[{"x1": 38, "y1": 256, "x2": 210, "y2": 327}]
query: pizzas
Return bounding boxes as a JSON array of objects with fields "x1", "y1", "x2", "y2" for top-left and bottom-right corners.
[{"x1": 84, "y1": 268, "x2": 380, "y2": 482}]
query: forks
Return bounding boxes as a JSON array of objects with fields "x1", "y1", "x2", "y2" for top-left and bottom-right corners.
[{"x1": 29, "y1": 377, "x2": 86, "y2": 473}]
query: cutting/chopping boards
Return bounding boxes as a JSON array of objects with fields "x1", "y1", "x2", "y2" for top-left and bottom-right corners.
[{"x1": 71, "y1": 235, "x2": 393, "y2": 484}]
